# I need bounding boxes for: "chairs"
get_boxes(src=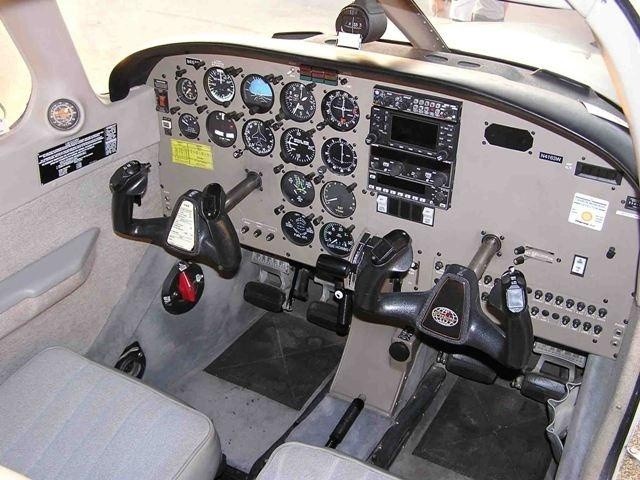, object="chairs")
[0,346,223,479]
[256,440,402,479]
